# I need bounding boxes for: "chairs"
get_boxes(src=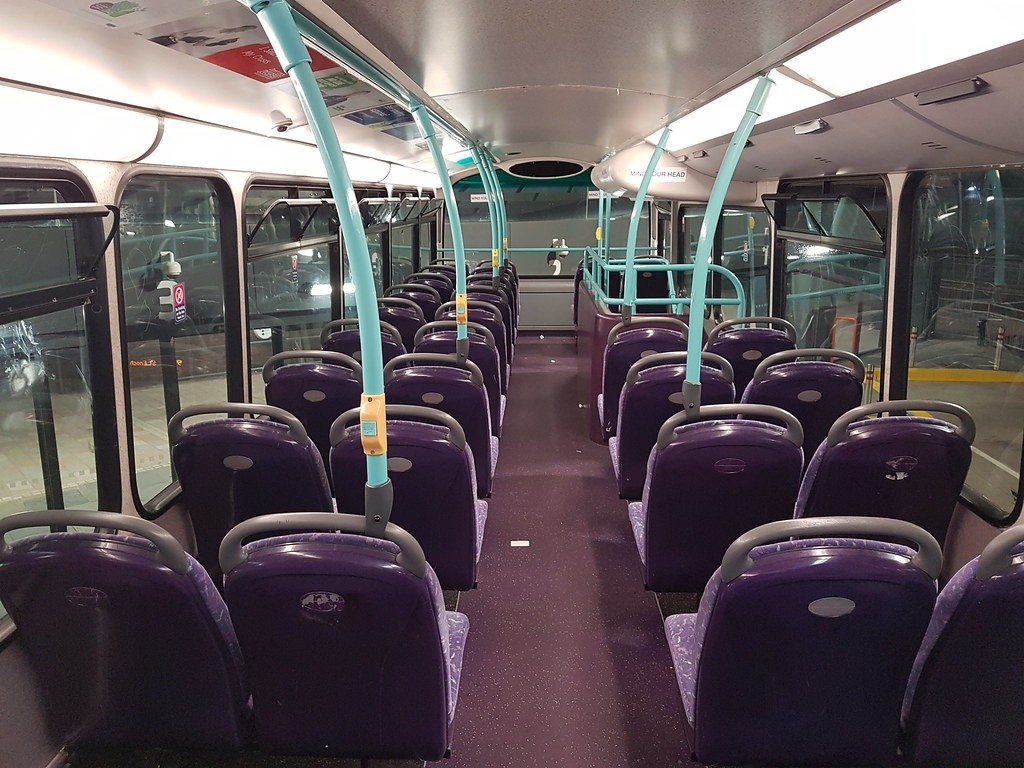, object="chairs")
[0,257,1023,767]
